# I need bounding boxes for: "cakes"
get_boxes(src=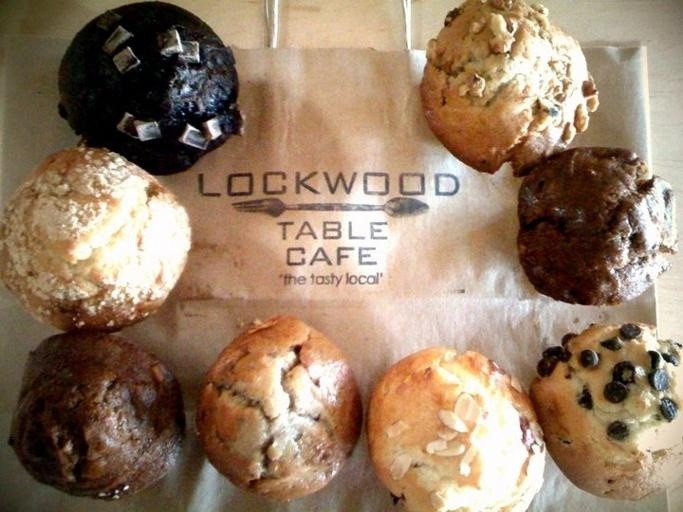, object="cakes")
[366,348,545,512]
[58,2,239,175]
[517,146,679,305]
[0,145,191,332]
[195,316,362,500]
[421,0,599,176]
[10,332,185,500]
[529,323,683,500]
